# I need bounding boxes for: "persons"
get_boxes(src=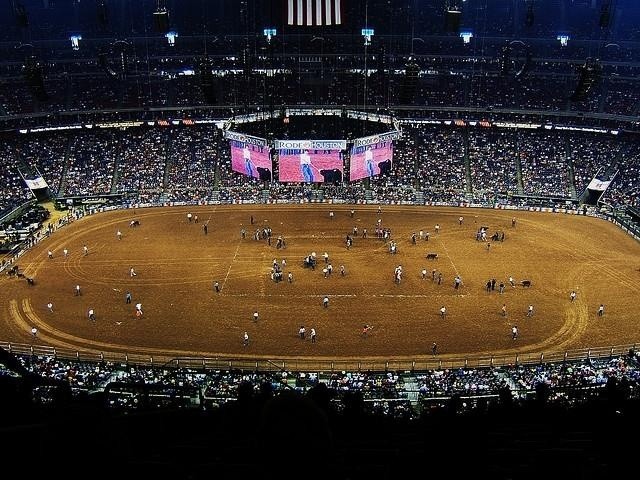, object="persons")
[298,326,307,339]
[511,325,517,340]
[135,303,143,316]
[309,329,316,343]
[598,304,603,316]
[75,284,82,297]
[87,307,96,323]
[1,349,639,478]
[431,341,439,355]
[570,291,576,302]
[440,306,447,319]
[324,296,328,307]
[253,311,258,323]
[1,108,639,291]
[126,292,131,304]
[364,323,374,336]
[135,309,141,321]
[242,331,248,346]
[361,325,367,336]
[30,327,38,339]
[501,304,506,316]
[527,305,534,317]
[48,302,56,312]
